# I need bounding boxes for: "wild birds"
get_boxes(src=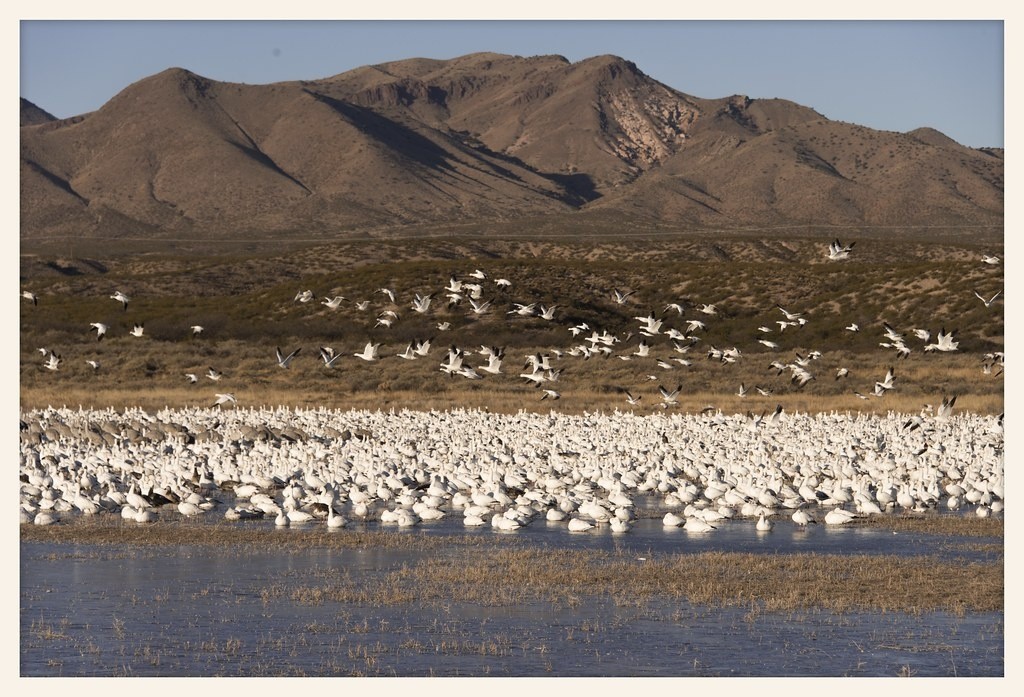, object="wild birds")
[320,347,344,370]
[980,255,1000,265]
[190,325,204,337]
[972,288,1004,309]
[539,389,562,401]
[317,347,335,363]
[824,238,856,261]
[109,290,129,313]
[89,322,111,342]
[320,296,351,311]
[20,290,40,306]
[658,385,683,403]
[980,351,1004,378]
[854,392,869,400]
[205,366,222,382]
[293,289,317,303]
[354,300,370,312]
[754,385,774,398]
[624,390,641,405]
[877,366,897,396]
[208,392,237,409]
[869,384,887,397]
[184,373,198,384]
[276,346,302,370]
[734,382,752,399]
[20,395,1004,533]
[36,347,51,357]
[40,350,63,371]
[371,267,961,389]
[128,322,145,338]
[85,360,100,369]
[353,340,386,362]
